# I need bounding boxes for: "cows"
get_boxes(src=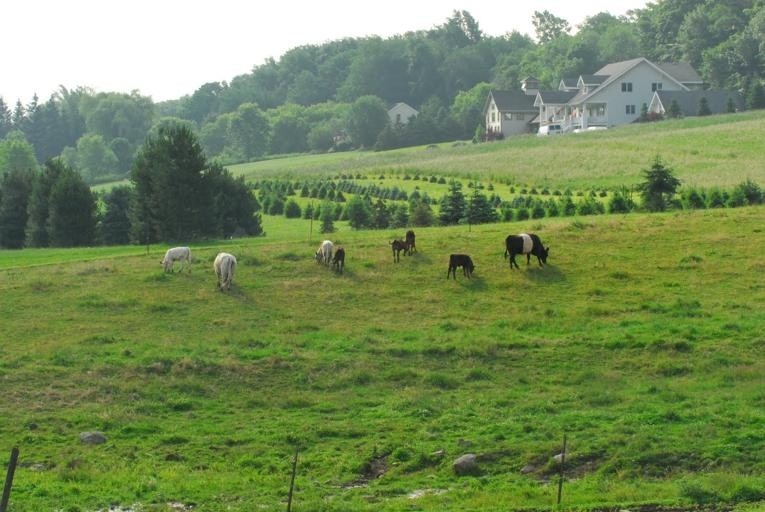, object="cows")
[312,240,346,275]
[158,245,193,274]
[211,251,237,290]
[386,230,417,264]
[502,232,550,270]
[446,253,476,281]
